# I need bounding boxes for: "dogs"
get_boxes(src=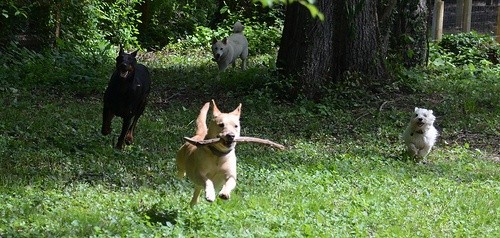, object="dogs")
[212,21,248,73]
[176,99,244,210]
[404,107,438,160]
[101,44,151,152]
